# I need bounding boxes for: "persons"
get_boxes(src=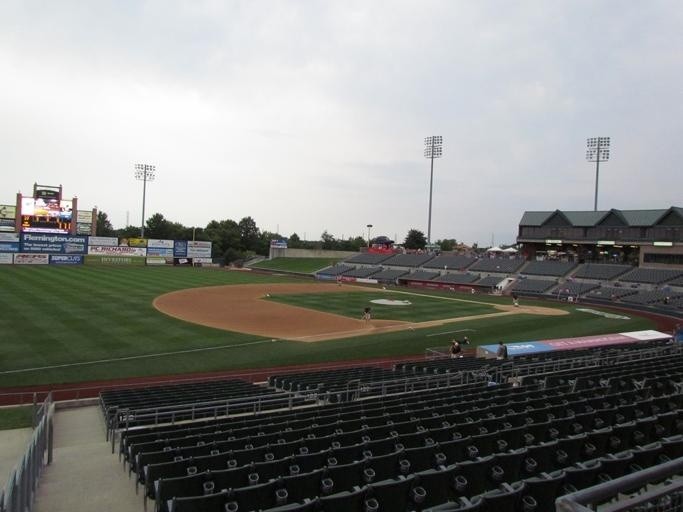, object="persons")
[495,341,507,360]
[362,304,372,329]
[447,341,462,359]
